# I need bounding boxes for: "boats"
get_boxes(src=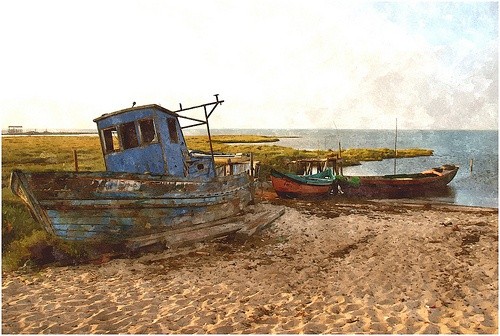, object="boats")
[9,94,261,267]
[268,167,333,200]
[335,163,459,199]
